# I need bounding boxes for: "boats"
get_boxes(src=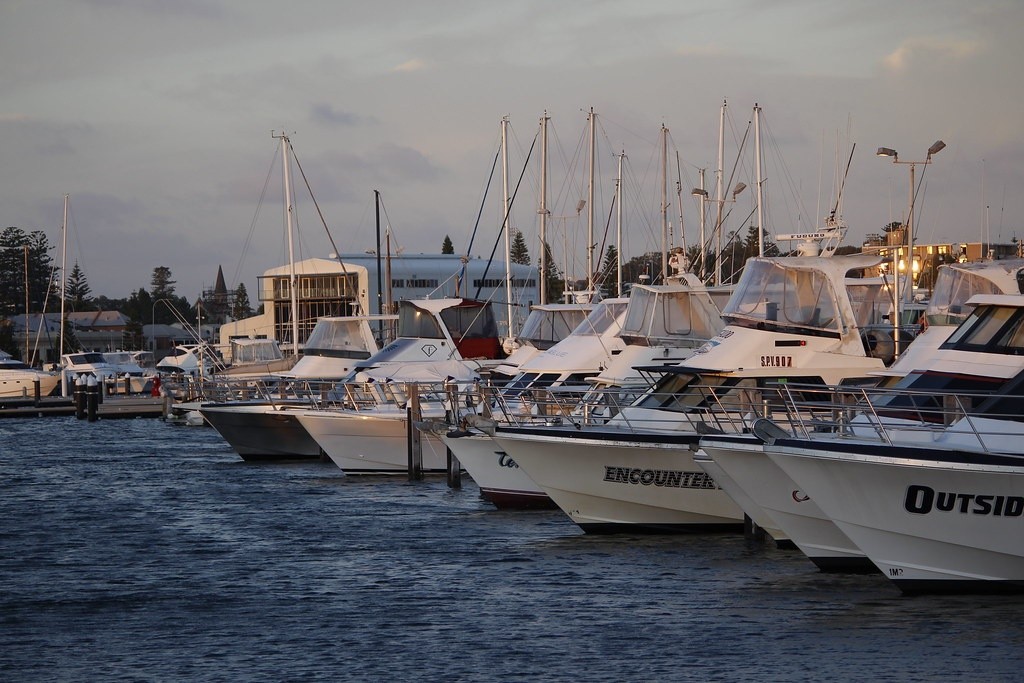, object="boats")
[756,287,1023,597]
[916,256,1024,322]
[415,297,631,510]
[0,348,60,399]
[199,314,374,464]
[691,447,797,553]
[478,253,913,534]
[287,299,475,480]
[697,295,1023,576]
[62,342,224,381]
